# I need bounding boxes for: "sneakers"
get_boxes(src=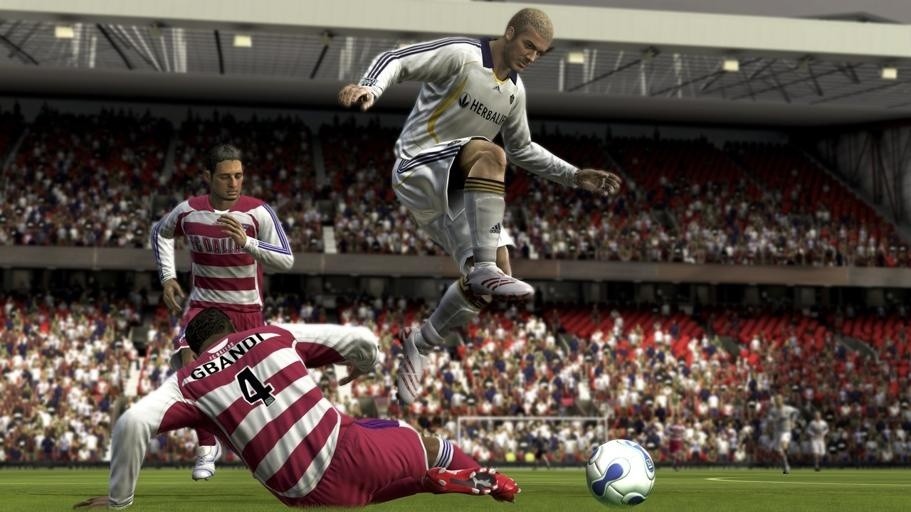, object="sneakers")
[192,435,222,481]
[398,327,428,405]
[424,467,522,503]
[469,263,534,300]
